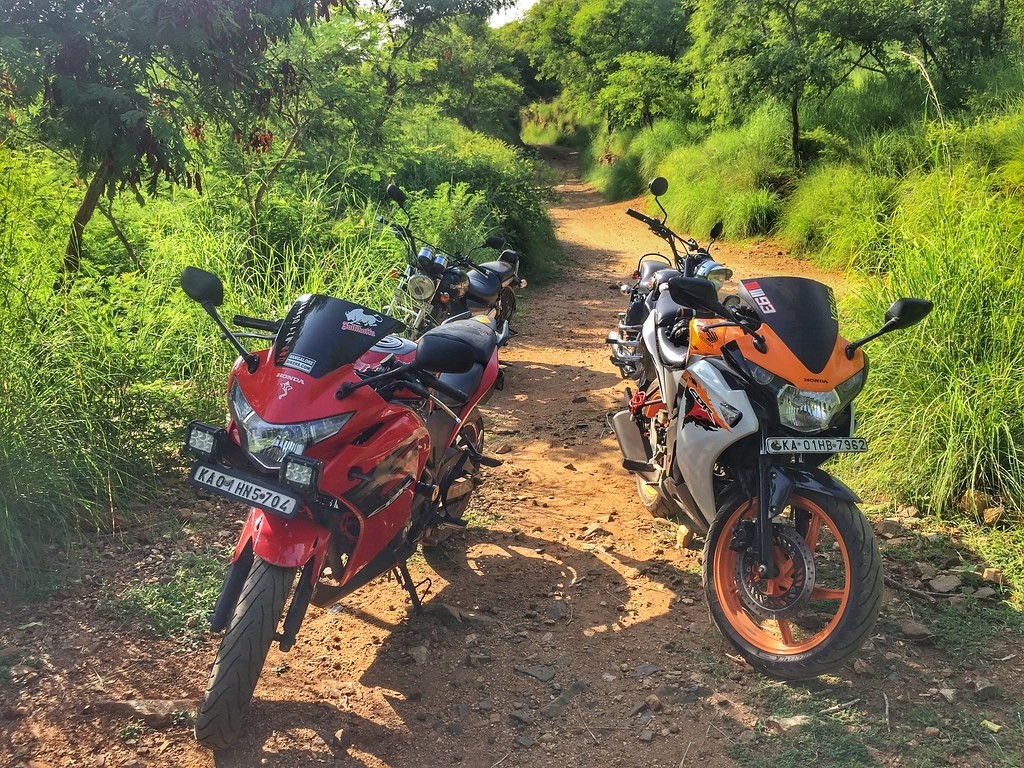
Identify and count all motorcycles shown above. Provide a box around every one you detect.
[376,184,527,368]
[607,177,733,381]
[613,275,940,683]
[179,265,509,754]
